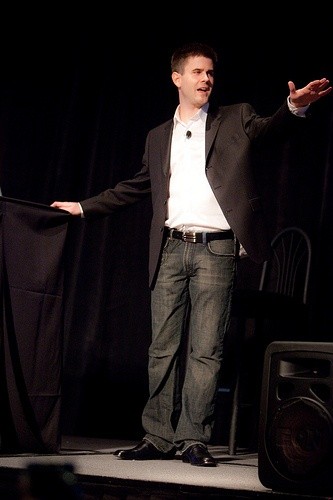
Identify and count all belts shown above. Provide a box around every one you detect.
[163,230,234,243]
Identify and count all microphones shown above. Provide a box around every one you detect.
[186,131,191,137]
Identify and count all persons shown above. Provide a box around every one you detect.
[50,43,332,467]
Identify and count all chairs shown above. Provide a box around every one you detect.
[218,225,313,457]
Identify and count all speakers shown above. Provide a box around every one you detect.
[258,342,333,497]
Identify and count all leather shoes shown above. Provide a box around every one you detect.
[112,439,176,460]
[182,444,218,466]
